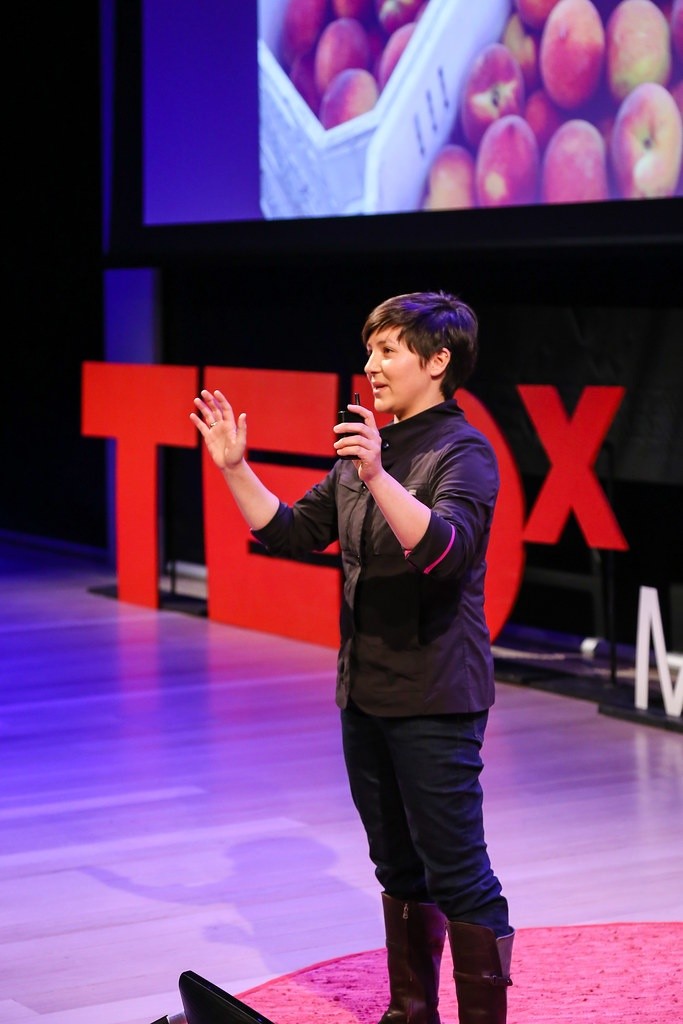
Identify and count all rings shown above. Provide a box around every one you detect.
[210,421,217,427]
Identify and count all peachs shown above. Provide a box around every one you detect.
[419,0,683,210]
[284,0,432,130]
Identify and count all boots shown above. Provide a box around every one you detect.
[445,920,515,1024]
[377,891,449,1024]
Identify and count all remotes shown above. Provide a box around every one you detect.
[337,392,365,460]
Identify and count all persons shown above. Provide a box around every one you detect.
[191,288,516,1024]
